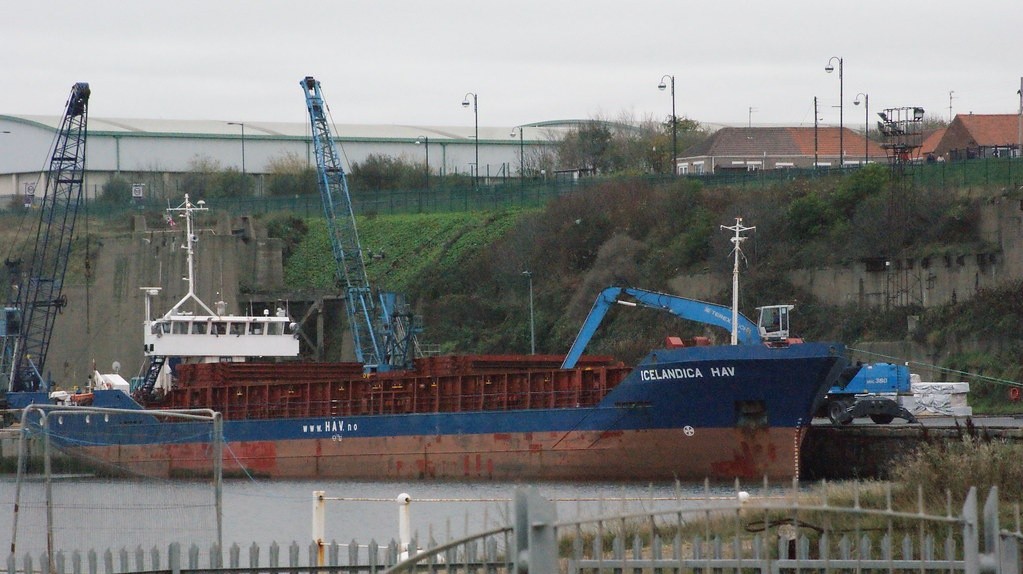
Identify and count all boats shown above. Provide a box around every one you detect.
[6,77,853,486]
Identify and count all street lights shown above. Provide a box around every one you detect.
[825,56,843,164]
[658,74,676,175]
[854,93,868,164]
[510,126,524,184]
[228,123,245,198]
[462,92,478,186]
[414,136,429,205]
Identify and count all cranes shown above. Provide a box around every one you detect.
[298,76,423,375]
[1,82,92,395]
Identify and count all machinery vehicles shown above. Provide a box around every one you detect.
[560,286,920,424]
